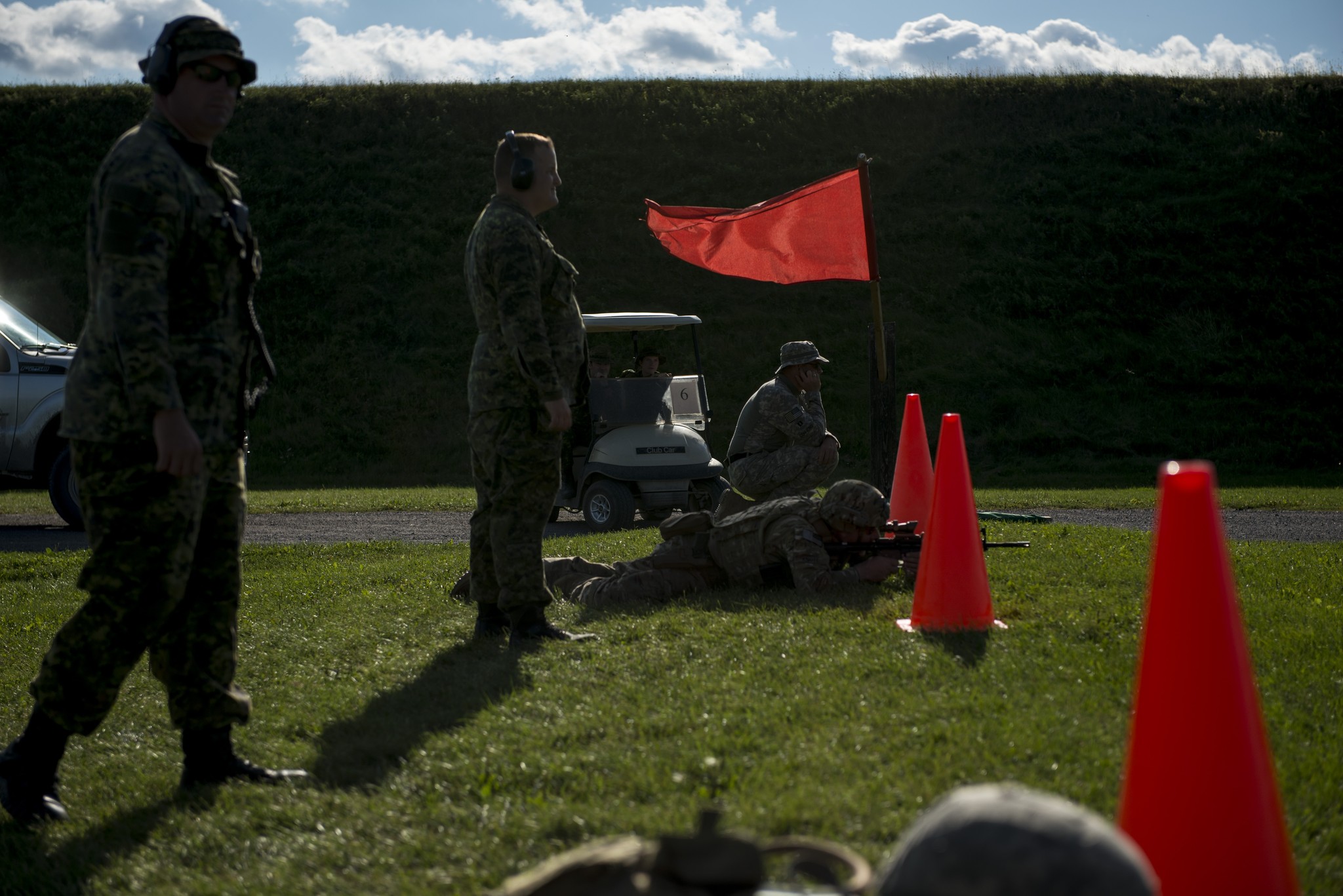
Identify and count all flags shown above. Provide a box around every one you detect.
[645,169,871,284]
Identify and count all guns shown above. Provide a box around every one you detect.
[830,521,1030,573]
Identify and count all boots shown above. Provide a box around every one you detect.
[475,605,511,637]
[715,489,765,522]
[0,712,73,824]
[508,605,601,652]
[180,725,308,790]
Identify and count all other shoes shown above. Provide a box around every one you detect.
[450,568,471,598]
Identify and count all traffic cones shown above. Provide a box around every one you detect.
[897,411,1016,636]
[1119,459,1305,895]
[886,393,941,549]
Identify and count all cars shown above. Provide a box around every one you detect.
[0,295,86,533]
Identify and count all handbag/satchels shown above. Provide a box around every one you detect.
[659,511,715,542]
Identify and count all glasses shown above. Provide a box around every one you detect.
[187,61,244,88]
[857,525,875,535]
[806,362,820,370]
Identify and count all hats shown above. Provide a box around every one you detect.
[146,16,256,86]
[620,370,635,378]
[635,347,667,366]
[775,341,829,373]
[588,345,614,364]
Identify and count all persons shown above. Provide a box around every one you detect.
[0,14,308,826]
[726,341,840,501]
[561,346,673,500]
[465,130,587,654]
[501,785,1159,896]
[451,479,920,611]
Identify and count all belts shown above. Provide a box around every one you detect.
[723,452,752,468]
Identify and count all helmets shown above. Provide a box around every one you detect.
[819,479,891,532]
[872,781,1159,896]
